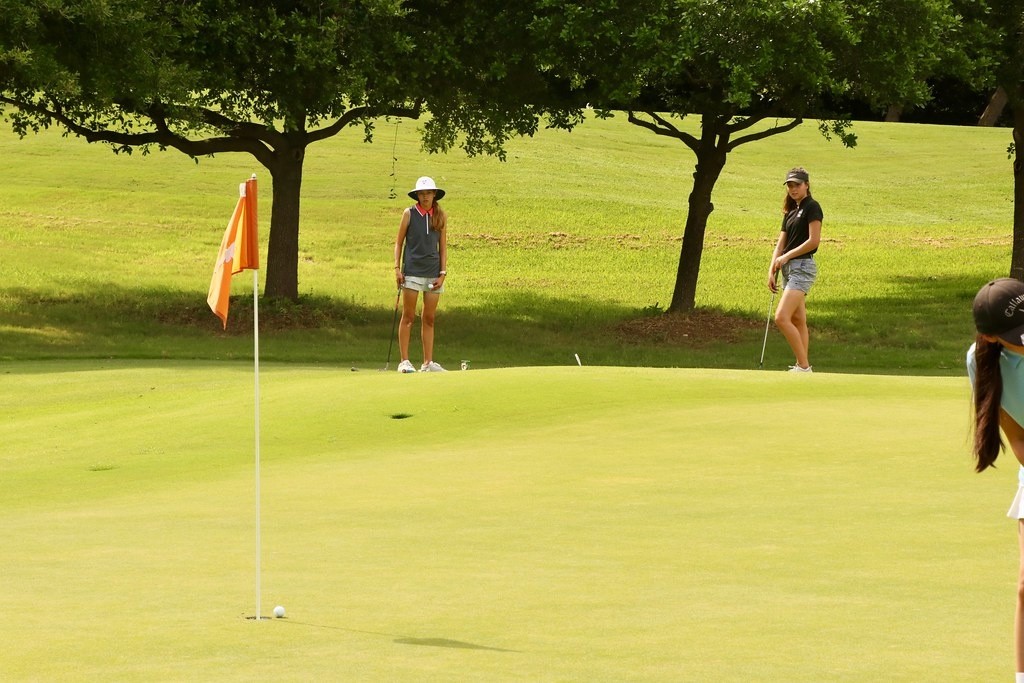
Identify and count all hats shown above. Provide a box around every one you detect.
[783,171,809,185]
[408,176,445,202]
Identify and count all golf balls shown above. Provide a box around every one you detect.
[273,606,285,618]
[427,284,434,289]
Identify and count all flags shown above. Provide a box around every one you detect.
[207,178,259,330]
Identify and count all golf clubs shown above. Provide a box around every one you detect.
[376,245,407,372]
[753,265,780,370]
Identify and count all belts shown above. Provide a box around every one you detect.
[795,255,813,259]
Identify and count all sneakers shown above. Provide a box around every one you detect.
[788,363,812,371]
[398,360,416,373]
[421,361,447,371]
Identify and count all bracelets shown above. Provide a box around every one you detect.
[395,267,400,269]
[440,271,447,276]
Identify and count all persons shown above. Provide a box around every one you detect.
[395,176,448,373]
[967,277,1024,683]
[767,167,823,373]
